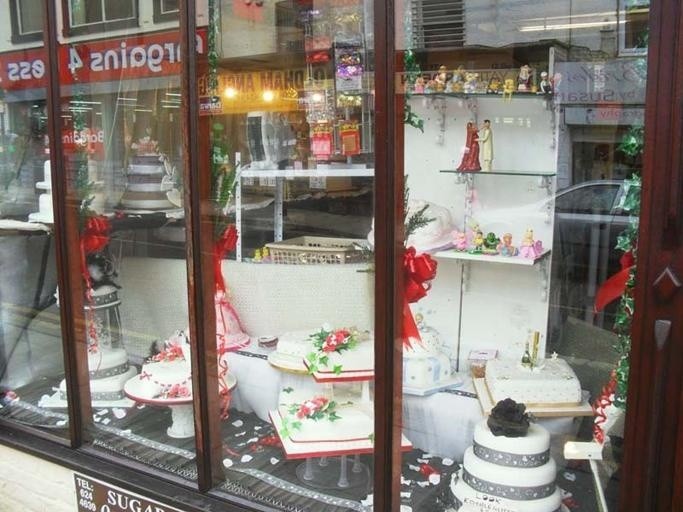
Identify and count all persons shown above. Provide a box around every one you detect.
[456,120,491,171]
[451,222,543,260]
[411,61,552,96]
[473,119,494,172]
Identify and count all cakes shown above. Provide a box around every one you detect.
[306,323,374,373]
[61,348,137,402]
[449,397,571,511]
[119,140,177,210]
[367,198,463,257]
[140,356,193,398]
[484,356,583,408]
[39,158,107,215]
[278,395,374,442]
[273,328,312,368]
[401,314,451,388]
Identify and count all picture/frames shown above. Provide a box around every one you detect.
[61,0,139,38]
[9,0,44,44]
[153,0,181,23]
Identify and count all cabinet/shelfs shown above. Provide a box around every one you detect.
[409,85,555,265]
[233,152,375,264]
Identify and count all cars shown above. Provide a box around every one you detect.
[469,177,660,279]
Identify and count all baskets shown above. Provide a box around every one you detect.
[265,236,371,265]
